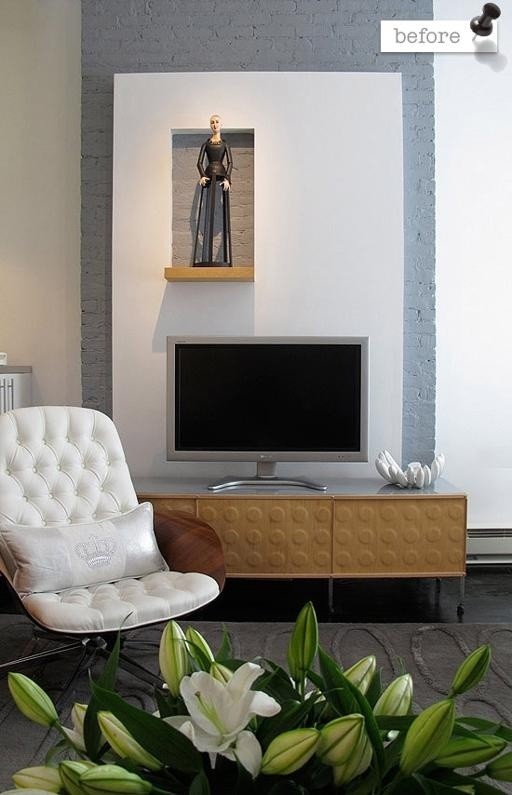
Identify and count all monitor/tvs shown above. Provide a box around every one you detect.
[166,335,370,491]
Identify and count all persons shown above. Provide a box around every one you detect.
[193,115,235,267]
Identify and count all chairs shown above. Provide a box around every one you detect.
[0,404,227,715]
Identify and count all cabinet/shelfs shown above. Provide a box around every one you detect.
[0,365,32,414]
[155,492,470,616]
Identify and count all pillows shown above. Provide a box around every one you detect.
[1,500,173,596]
